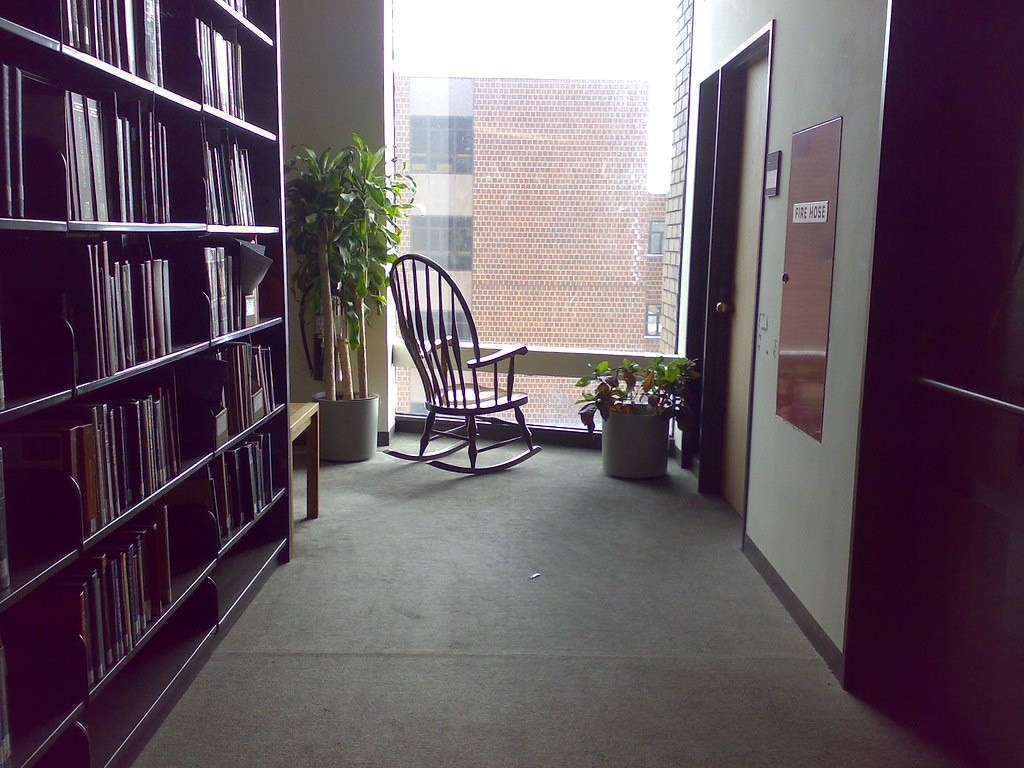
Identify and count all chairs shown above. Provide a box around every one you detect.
[383,253,545,474]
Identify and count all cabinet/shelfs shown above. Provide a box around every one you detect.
[0,0,291,768]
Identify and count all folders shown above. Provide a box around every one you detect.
[84,97,108,222]
[70,91,95,222]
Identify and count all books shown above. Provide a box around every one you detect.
[82,0,275,692]
[0,66,25,767]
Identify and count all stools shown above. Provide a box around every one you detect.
[288,402,323,560]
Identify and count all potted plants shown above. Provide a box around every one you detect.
[574,355,701,480]
[283,133,419,464]
